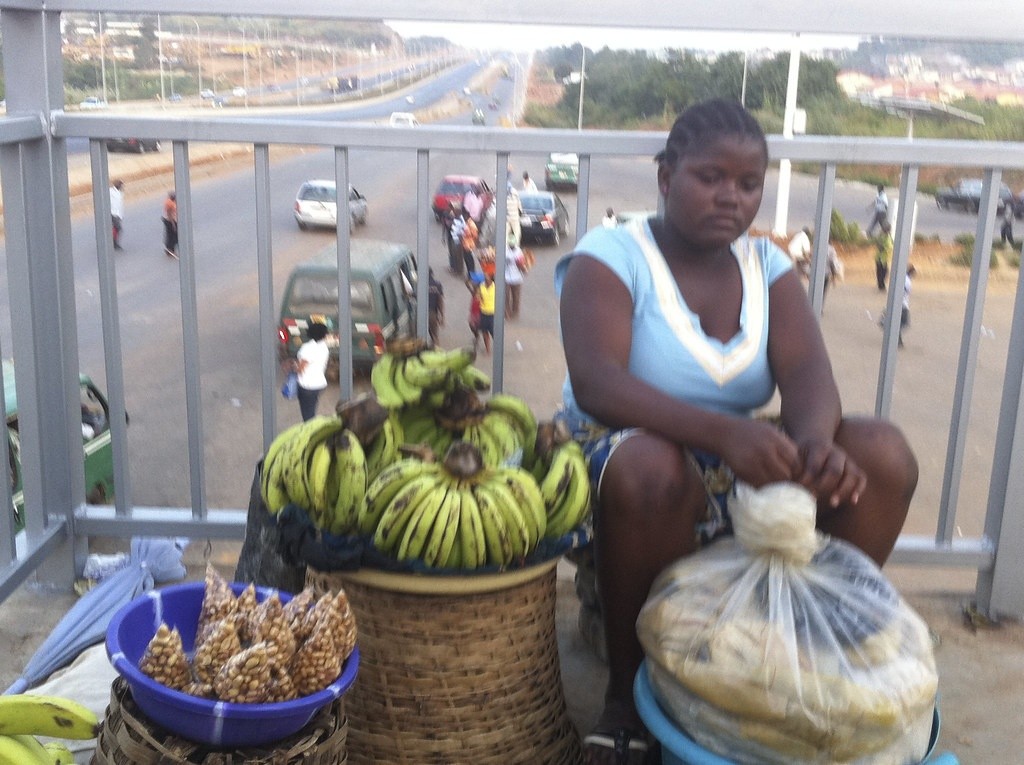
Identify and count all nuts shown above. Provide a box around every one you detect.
[137,568,357,704]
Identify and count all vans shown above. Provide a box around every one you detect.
[3,358,130,532]
[390,112,418,127]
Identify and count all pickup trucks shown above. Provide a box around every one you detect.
[78,97,105,109]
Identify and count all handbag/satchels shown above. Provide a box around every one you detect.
[282,370,298,401]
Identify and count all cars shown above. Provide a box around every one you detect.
[106,137,160,153]
[462,87,499,125]
[520,191,569,246]
[201,87,246,106]
[432,174,493,221]
[169,94,181,102]
[294,179,368,232]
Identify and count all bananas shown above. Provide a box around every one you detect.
[0,694,99,765]
[256,342,595,573]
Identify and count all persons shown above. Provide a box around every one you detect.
[545,101,920,765]
[602,208,616,227]
[162,192,179,259]
[293,322,329,424]
[110,181,124,250]
[822,239,840,316]
[898,264,916,349]
[1001,199,1015,249]
[788,227,812,283]
[427,167,538,356]
[866,183,888,237]
[874,222,894,293]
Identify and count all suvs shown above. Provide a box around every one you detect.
[935,179,1024,218]
[279,237,420,368]
[546,152,579,188]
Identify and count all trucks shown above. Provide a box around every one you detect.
[326,76,358,93]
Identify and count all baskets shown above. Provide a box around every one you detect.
[303,559,588,765]
[91,680,348,765]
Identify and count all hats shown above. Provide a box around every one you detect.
[468,271,484,285]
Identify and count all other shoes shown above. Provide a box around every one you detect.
[113,243,122,250]
[163,250,177,260]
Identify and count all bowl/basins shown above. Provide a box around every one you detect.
[103,583,360,750]
[632,660,939,765]
